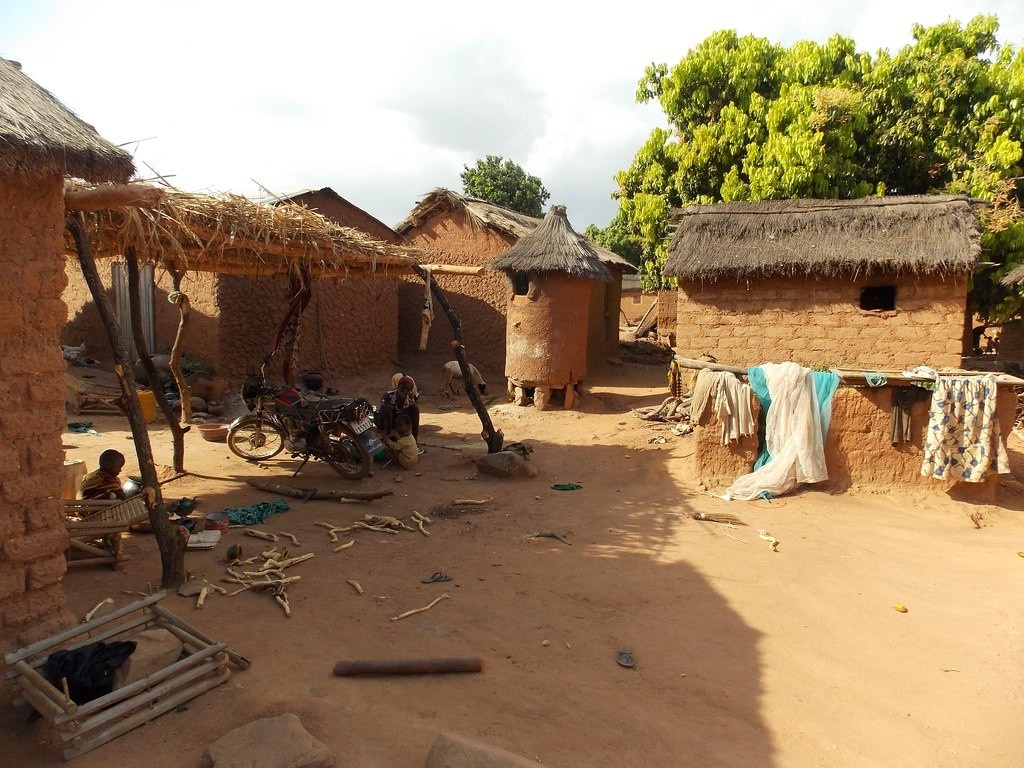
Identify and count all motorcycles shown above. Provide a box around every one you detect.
[226,352,386,481]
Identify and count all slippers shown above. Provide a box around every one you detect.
[421,572,453,583]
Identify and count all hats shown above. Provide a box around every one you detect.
[397,376,414,391]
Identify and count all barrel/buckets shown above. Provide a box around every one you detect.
[136,390,157,424]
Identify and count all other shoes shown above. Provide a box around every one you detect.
[417,445,428,453]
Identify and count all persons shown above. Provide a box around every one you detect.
[74,449,127,520]
[377,376,419,443]
[383,414,419,471]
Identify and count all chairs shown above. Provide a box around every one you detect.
[63,492,149,571]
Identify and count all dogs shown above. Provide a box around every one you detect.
[442,360,486,396]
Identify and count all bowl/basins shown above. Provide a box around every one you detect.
[130,519,153,534]
[205,512,228,534]
[196,423,230,442]
